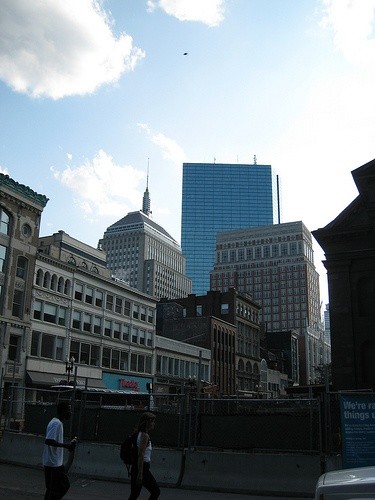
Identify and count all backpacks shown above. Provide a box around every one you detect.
[119,431,150,464]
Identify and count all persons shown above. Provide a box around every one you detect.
[42,399,78,500]
[128,412,161,500]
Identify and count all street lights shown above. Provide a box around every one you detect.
[64,355,75,384]
